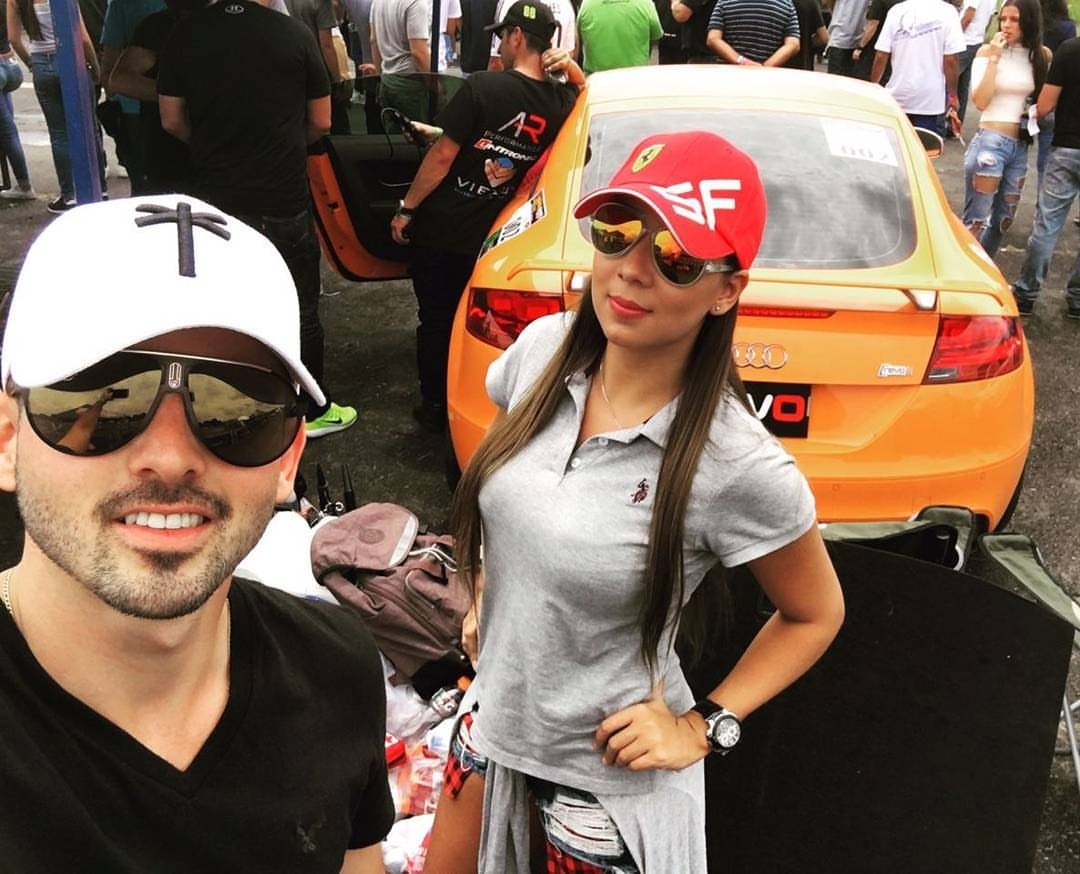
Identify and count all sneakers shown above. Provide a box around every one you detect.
[1,185,36,200]
[48,193,77,214]
[305,401,358,440]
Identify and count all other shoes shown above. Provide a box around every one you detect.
[118,164,130,179]
[1067,307,1080,319]
[413,398,445,434]
[105,167,111,180]
[1009,284,1034,314]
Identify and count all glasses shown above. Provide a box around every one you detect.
[589,202,738,288]
[11,344,311,466]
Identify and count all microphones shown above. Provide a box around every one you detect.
[400,114,429,148]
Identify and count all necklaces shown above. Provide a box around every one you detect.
[600,357,626,430]
[4,566,15,615]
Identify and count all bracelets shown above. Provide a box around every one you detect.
[396,214,412,219]
[737,55,743,64]
[989,56,998,64]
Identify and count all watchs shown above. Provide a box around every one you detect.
[690,695,743,756]
[395,199,416,216]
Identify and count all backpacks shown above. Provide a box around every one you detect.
[310,503,476,688]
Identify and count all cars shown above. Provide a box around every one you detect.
[304,64,1034,569]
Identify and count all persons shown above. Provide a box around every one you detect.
[963,0,1080,320]
[424,133,845,874]
[97,0,357,437]
[0,0,999,212]
[0,195,396,874]
[391,0,587,434]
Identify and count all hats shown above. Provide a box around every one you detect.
[574,132,766,270]
[483,0,562,49]
[1,192,326,406]
